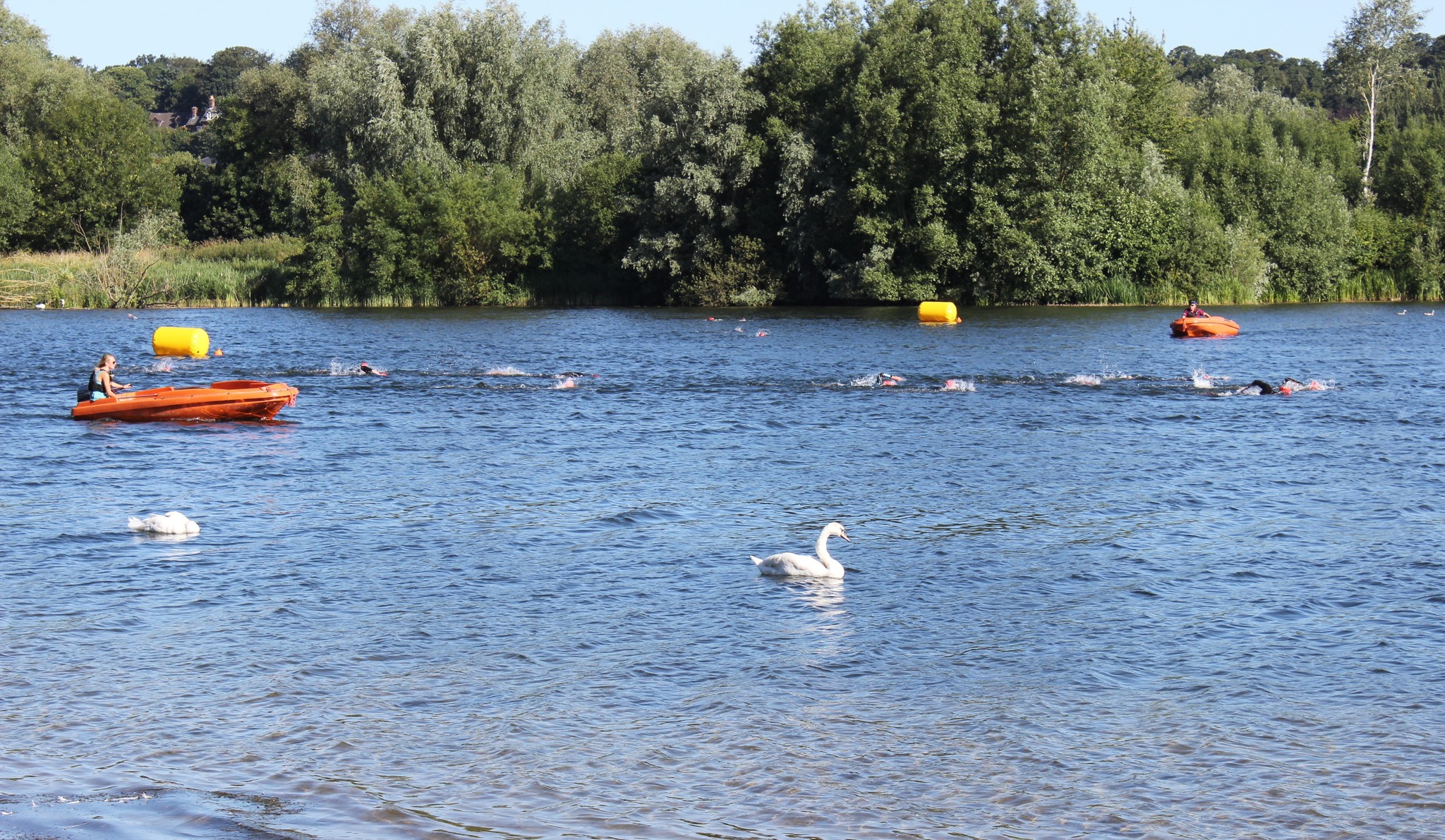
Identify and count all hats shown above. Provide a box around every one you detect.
[1189,300,1198,305]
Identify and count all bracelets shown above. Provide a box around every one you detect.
[122,385,124,389]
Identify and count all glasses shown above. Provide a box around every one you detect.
[107,361,116,364]
[1189,305,1195,306]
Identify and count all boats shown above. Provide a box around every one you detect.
[1169,316,1240,338]
[71,379,300,422]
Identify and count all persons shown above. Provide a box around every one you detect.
[1182,301,1211,319]
[543,372,602,379]
[361,363,387,375]
[759,332,769,336]
[1241,379,1291,396]
[709,317,714,321]
[1283,377,1324,392]
[88,354,132,401]
[853,373,902,388]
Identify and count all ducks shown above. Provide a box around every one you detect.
[1395,309,1407,315]
[1423,310,1435,315]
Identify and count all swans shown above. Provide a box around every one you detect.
[128,511,200,534]
[750,522,850,580]
